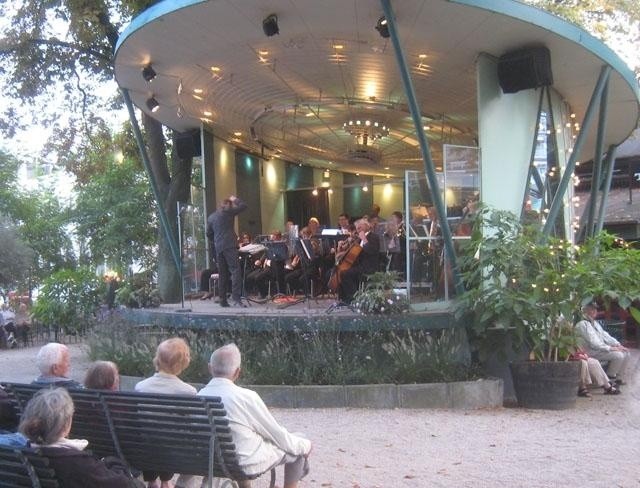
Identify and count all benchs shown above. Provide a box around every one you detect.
[600,360,610,367]
[0,381,276,488]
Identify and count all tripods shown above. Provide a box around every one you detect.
[325,270,357,314]
[295,258,318,305]
[239,254,262,306]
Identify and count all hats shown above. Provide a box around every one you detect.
[587,301,601,308]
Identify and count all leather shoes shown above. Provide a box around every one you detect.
[234,301,247,308]
[220,300,230,307]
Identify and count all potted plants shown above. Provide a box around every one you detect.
[451,200,640,413]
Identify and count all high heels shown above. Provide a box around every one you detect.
[200,295,211,300]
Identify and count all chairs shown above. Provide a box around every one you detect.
[208,216,471,313]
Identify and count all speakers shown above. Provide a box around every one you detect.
[497,47,554,93]
[176,129,201,159]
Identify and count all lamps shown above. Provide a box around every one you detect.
[145,97,161,113]
[262,14,280,38]
[141,66,158,83]
[374,16,391,39]
[340,116,391,149]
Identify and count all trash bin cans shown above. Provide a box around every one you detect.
[597,319,626,347]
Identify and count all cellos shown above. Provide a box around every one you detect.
[327,221,378,289]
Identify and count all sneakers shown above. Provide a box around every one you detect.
[7,336,18,349]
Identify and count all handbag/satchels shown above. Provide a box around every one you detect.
[568,352,589,360]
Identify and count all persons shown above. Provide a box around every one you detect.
[572,294,631,385]
[198,341,312,488]
[552,309,621,398]
[29,342,78,389]
[84,359,172,488]
[137,338,199,488]
[243,191,481,306]
[104,275,118,311]
[18,388,145,488]
[207,196,248,308]
[0,292,31,348]
[200,268,217,300]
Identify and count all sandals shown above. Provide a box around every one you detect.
[579,388,592,398]
[603,386,621,395]
[609,377,625,388]
